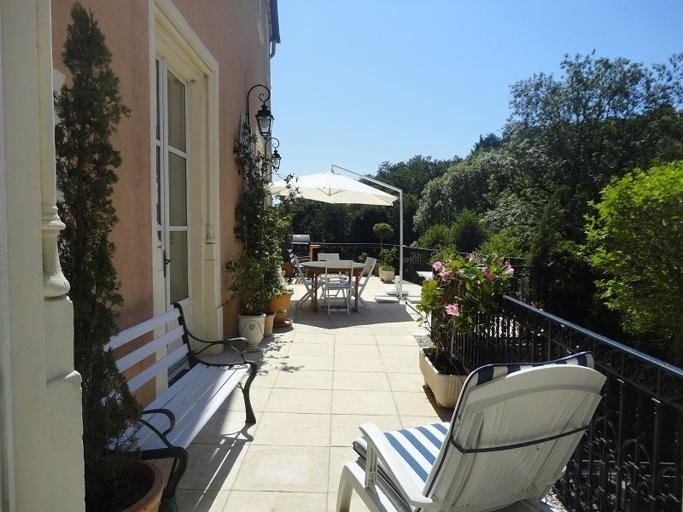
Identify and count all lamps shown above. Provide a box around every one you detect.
[245,84,275,139]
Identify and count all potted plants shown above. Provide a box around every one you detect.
[233,121,301,352]
[54,2,164,512]
[372,222,395,276]
[379,246,398,281]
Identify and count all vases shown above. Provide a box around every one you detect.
[419,346,468,408]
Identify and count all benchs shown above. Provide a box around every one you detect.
[101,301,258,512]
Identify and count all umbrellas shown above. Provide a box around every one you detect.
[263,165,404,300]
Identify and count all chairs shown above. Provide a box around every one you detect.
[336,348,608,512]
[294,253,377,317]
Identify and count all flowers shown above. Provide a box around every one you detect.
[417,248,515,361]
